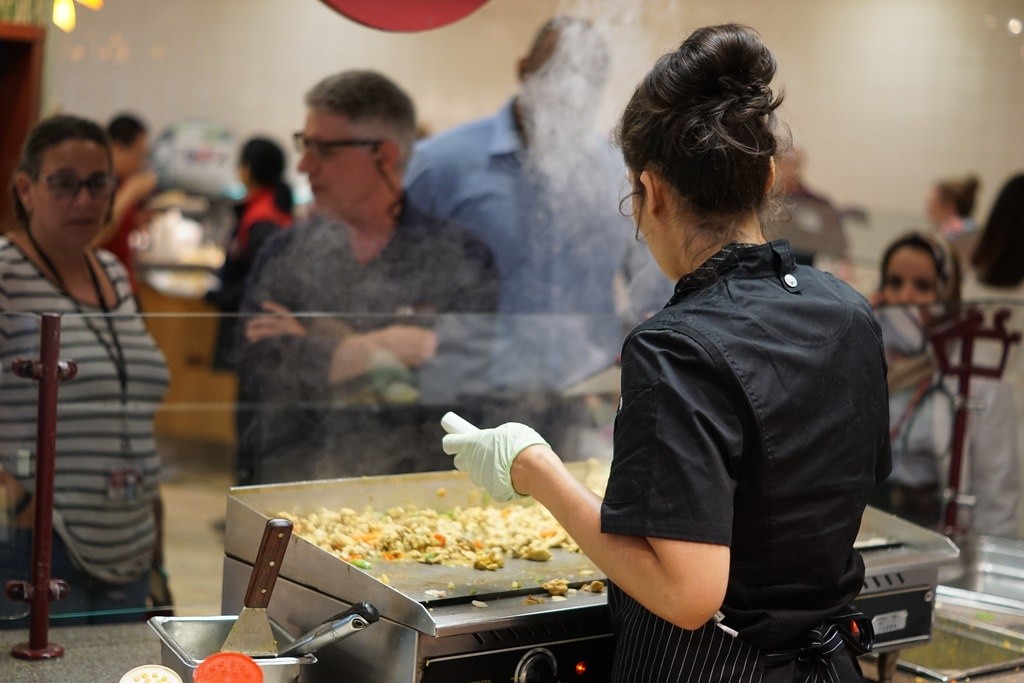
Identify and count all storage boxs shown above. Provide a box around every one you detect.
[147,614,317,683]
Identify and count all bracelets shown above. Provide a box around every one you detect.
[6,492,32,519]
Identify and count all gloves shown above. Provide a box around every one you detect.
[442,422,551,508]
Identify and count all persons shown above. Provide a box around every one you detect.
[93,115,160,288]
[398,18,674,466]
[877,229,963,305]
[762,150,847,257]
[199,139,293,529]
[227,71,498,491]
[0,117,173,621]
[441,24,892,682]
[928,178,978,240]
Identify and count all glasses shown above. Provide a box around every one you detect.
[31,168,117,200]
[293,133,379,160]
